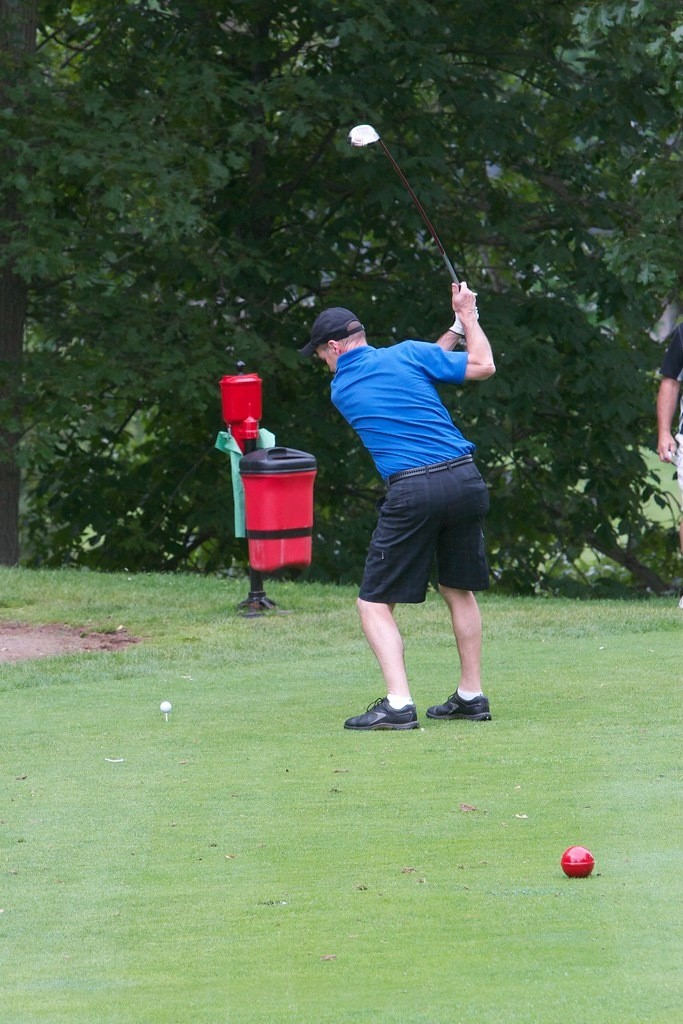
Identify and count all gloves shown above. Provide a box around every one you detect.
[449,293,479,335]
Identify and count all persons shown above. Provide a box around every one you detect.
[656,321,683,608]
[297,281,497,730]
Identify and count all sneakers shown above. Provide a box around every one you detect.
[344,696,419,730]
[426,686,492,721]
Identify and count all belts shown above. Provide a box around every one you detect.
[389,454,473,484]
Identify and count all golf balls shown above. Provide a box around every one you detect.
[159,702,173,712]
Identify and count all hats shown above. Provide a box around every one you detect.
[302,307,365,355]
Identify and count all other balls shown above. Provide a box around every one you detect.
[561,845,595,879]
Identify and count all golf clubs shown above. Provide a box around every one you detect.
[346,124,461,287]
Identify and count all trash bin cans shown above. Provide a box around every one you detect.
[238,446,318,573]
